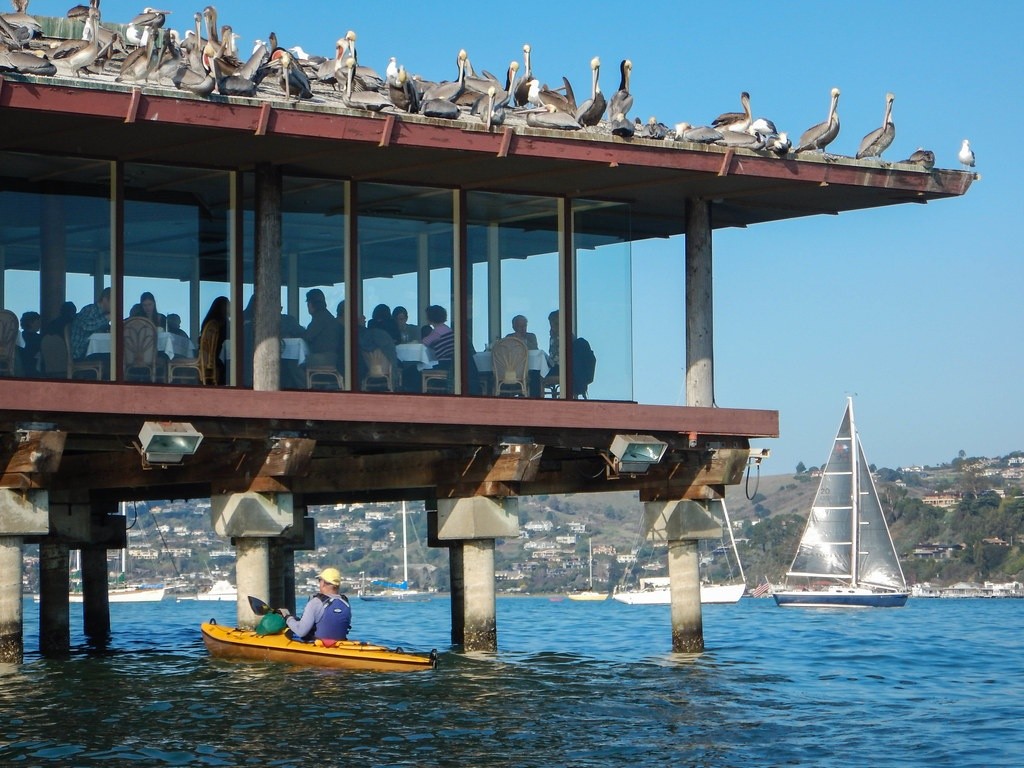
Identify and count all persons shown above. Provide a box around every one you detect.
[71,287,111,380]
[280,567,351,641]
[19,301,76,363]
[129,292,188,337]
[530,310,560,398]
[506,315,538,350]
[200,296,230,346]
[359,304,454,392]
[289,288,345,388]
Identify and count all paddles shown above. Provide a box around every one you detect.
[247,596,300,620]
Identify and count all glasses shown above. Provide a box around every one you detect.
[319,578,322,582]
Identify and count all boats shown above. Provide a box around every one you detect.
[195,580,238,602]
[201,618,438,671]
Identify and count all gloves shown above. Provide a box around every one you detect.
[277,608,291,617]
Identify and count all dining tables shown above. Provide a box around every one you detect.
[219,337,310,391]
[473,349,553,399]
[395,339,435,393]
[85,332,194,386]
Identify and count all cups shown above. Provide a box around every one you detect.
[401,331,409,341]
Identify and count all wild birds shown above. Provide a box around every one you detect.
[608,59,793,154]
[958,139,977,170]
[899,148,934,169]
[385,46,606,130]
[856,92,897,167]
[795,88,841,160]
[0,0,397,113]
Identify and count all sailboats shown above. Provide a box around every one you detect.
[613,498,746,604]
[68,501,165,602]
[359,501,439,603]
[773,397,911,607]
[568,538,609,600]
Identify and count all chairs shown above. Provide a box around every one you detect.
[0,309,595,399]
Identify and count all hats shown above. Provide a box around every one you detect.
[315,568,340,586]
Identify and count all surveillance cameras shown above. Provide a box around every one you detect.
[748,447,770,458]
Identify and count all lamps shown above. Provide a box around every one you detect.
[131,422,204,471]
[599,434,668,480]
[688,432,697,448]
